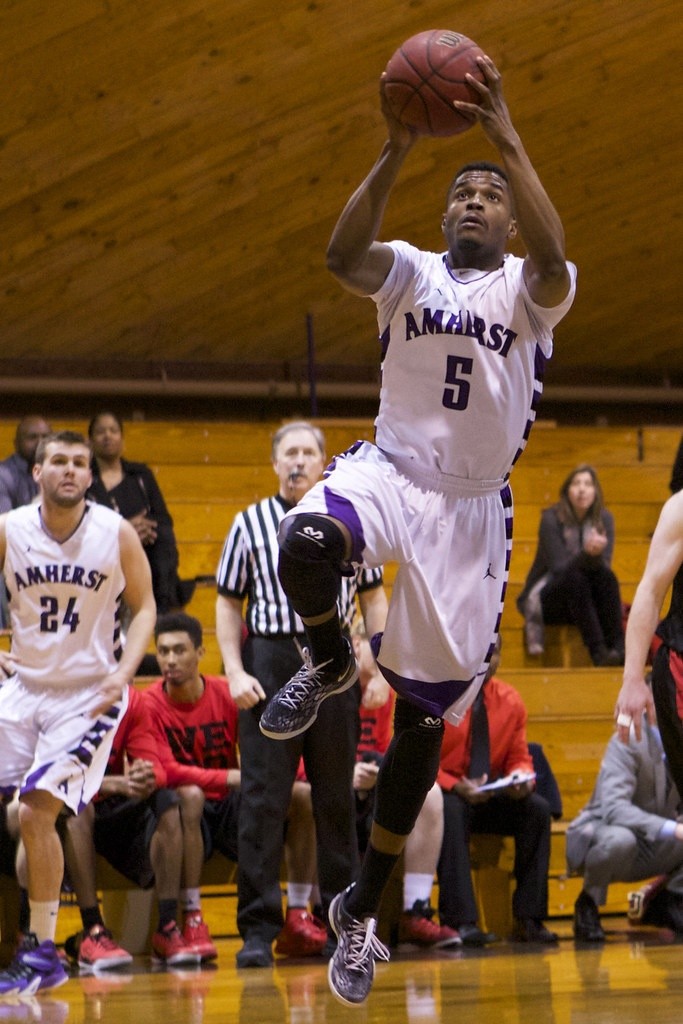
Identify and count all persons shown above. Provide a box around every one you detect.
[259,55,578,1006]
[0,408,683,1002]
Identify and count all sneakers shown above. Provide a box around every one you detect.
[258,635,357,741]
[0,931,68,1001]
[328,881,391,1008]
[400,898,462,948]
[276,904,328,958]
[77,923,134,970]
[182,909,219,963]
[150,919,201,965]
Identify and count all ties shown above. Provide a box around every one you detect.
[469,690,490,782]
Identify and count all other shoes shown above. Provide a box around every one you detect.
[236,935,272,967]
[514,916,556,944]
[457,923,495,946]
[640,885,683,934]
[572,897,605,940]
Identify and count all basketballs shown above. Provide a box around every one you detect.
[384,28,490,139]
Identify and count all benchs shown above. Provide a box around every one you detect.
[1,420,683,943]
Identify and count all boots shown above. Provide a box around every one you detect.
[582,626,627,667]
